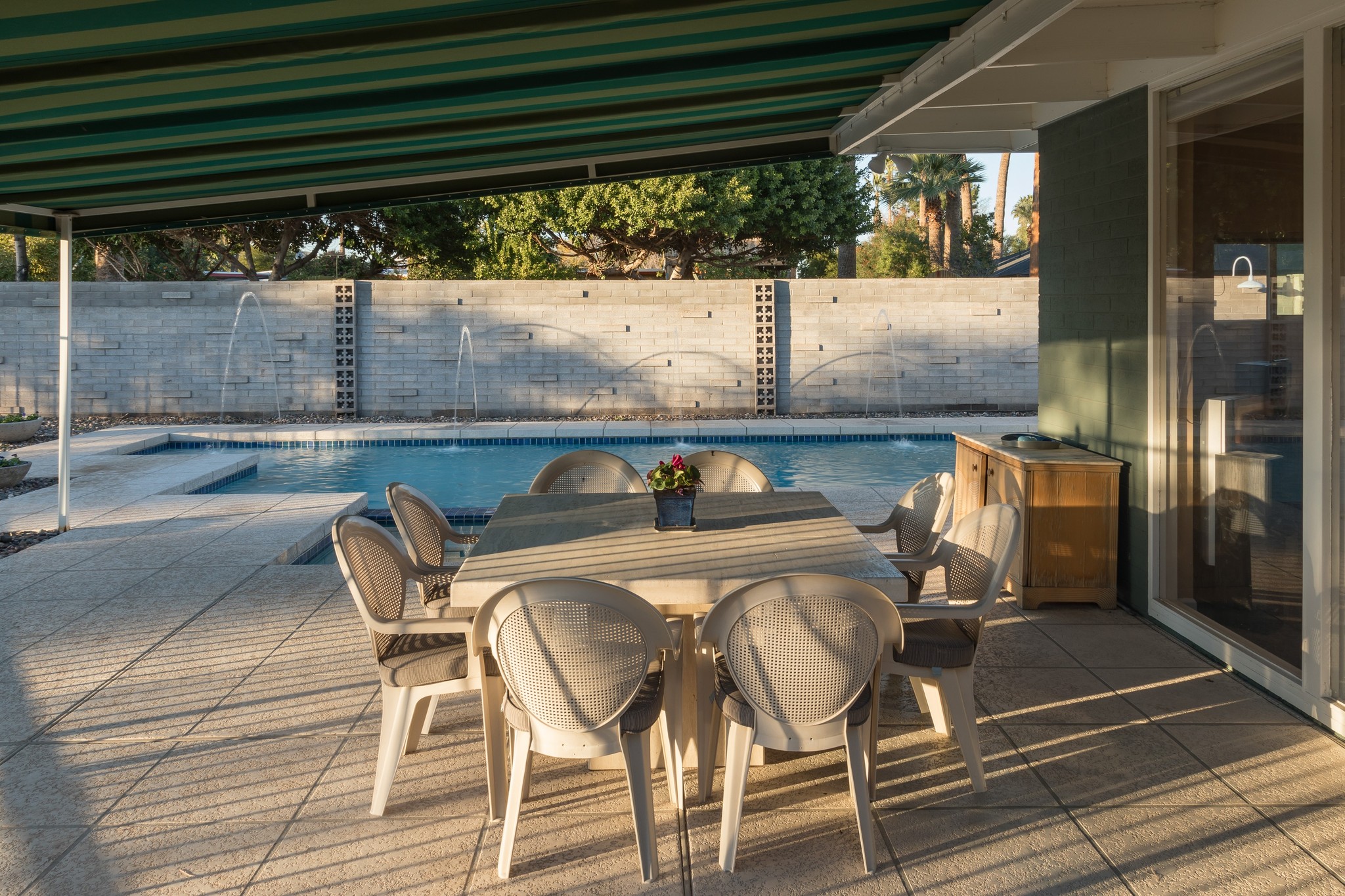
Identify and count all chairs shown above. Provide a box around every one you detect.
[386,481,481,619]
[683,449,775,493]
[694,574,905,875]
[527,447,650,494]
[862,502,1022,802]
[469,576,686,886]
[332,516,517,821]
[855,470,955,604]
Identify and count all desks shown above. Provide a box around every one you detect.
[449,491,907,770]
[951,431,1125,611]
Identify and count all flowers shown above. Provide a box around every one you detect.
[0,454,21,468]
[0,411,39,423]
[643,454,703,490]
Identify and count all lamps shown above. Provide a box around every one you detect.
[1231,256,1267,289]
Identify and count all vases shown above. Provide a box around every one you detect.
[0,416,43,444]
[0,461,33,489]
[652,485,698,532]
